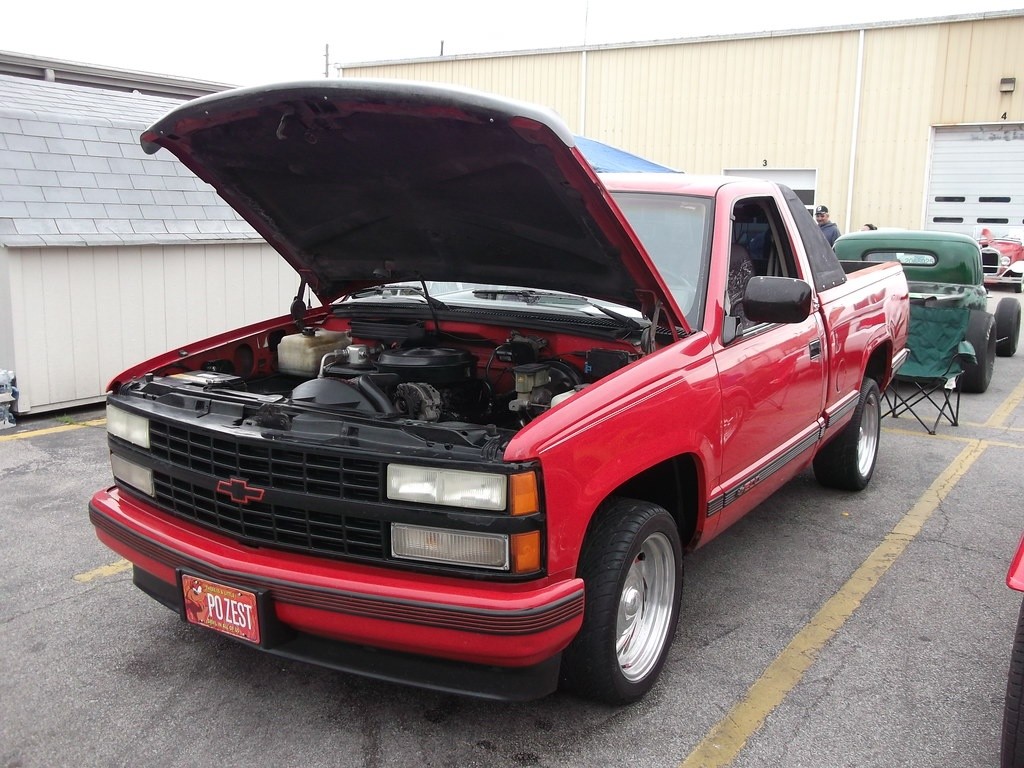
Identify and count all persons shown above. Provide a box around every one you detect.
[814,205,840,247]
[862,223,877,232]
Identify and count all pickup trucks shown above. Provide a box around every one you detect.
[86,78,912,714]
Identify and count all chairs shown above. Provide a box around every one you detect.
[879,304,979,434]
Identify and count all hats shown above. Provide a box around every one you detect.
[815,205,828,215]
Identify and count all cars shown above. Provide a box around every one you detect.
[827,228,1021,394]
[970,229,1024,292]
